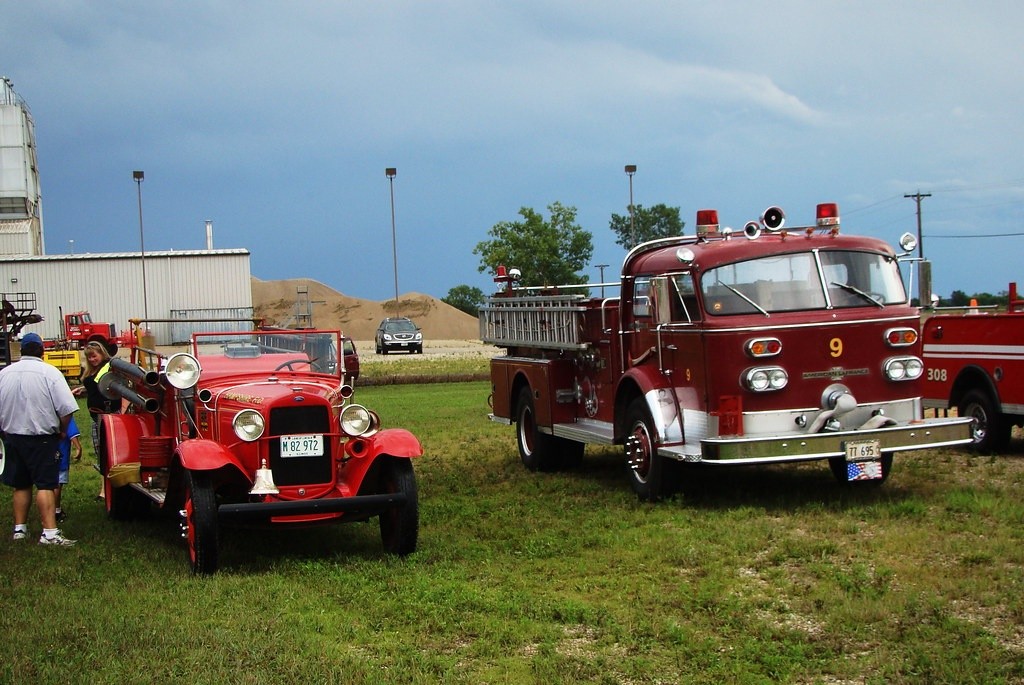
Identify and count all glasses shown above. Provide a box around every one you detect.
[84,342,100,347]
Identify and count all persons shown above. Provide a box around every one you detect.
[71,333,122,502]
[53,412,82,525]
[0,332,80,548]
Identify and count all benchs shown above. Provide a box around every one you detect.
[193,353,310,384]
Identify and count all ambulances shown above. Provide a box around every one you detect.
[907,281,1024,454]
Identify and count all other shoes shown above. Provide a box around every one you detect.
[14,529,31,541]
[55,510,67,522]
[37,532,79,547]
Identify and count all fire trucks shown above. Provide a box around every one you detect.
[477,199,985,506]
[92,313,427,570]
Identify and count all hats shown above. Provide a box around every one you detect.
[22,331,43,348]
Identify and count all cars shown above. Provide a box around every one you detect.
[9,299,356,392]
[375,317,423,356]
